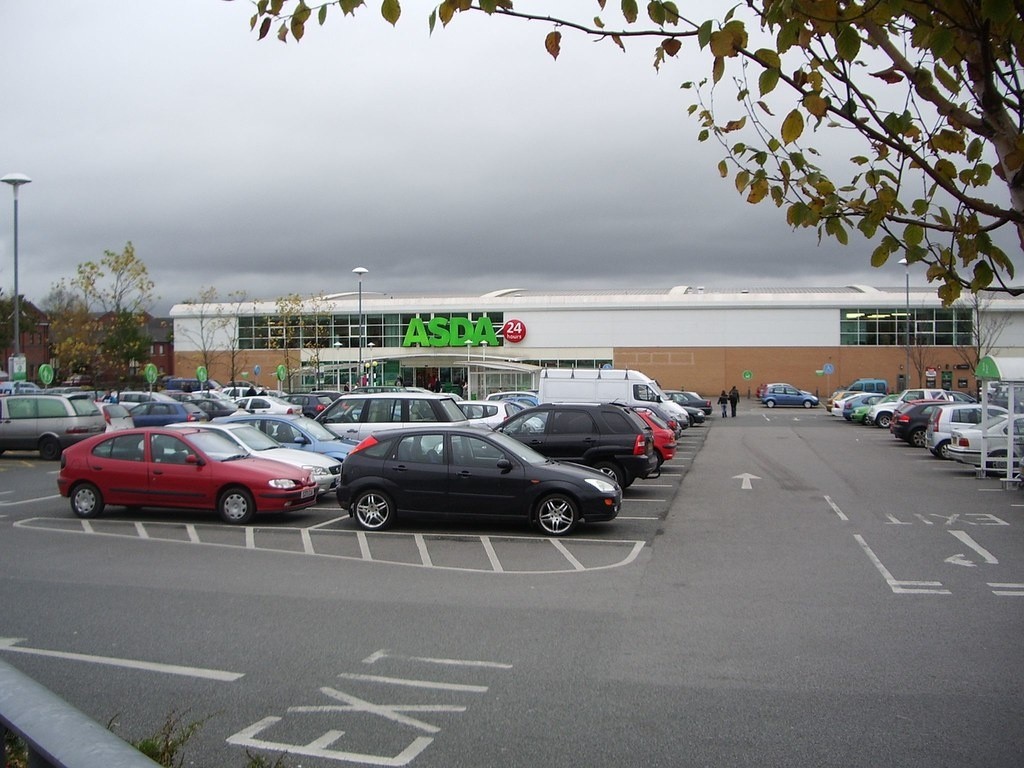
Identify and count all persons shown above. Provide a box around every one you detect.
[728,386,739,417]
[246,384,257,396]
[344,381,349,392]
[355,373,503,399]
[110,379,222,400]
[717,390,729,418]
[258,386,268,396]
[95,388,117,403]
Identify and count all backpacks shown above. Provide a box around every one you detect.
[731,390,737,400]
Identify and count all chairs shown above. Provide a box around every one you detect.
[152,444,169,463]
[170,440,189,464]
[277,424,291,442]
[427,448,442,463]
[410,405,422,420]
[967,412,978,424]
[267,425,275,437]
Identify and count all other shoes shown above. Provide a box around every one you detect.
[734,414,736,416]
[732,415,735,417]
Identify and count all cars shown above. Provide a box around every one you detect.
[831,391,883,421]
[947,414,1024,477]
[868,389,953,428]
[889,399,945,434]
[57,414,622,537]
[543,402,682,472]
[755,383,812,399]
[893,402,969,448]
[93,378,344,432]
[933,391,977,404]
[273,386,538,442]
[761,385,819,409]
[850,394,920,426]
[925,404,1008,460]
[842,395,887,421]
[650,390,713,427]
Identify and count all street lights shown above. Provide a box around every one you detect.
[464,339,488,401]
[0,173,32,356]
[333,267,376,392]
[897,258,918,388]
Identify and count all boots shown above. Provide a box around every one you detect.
[722,413,724,418]
[725,411,727,417]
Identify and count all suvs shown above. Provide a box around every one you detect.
[439,403,658,490]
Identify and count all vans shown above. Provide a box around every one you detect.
[0,393,107,459]
[847,379,889,396]
[538,363,691,429]
